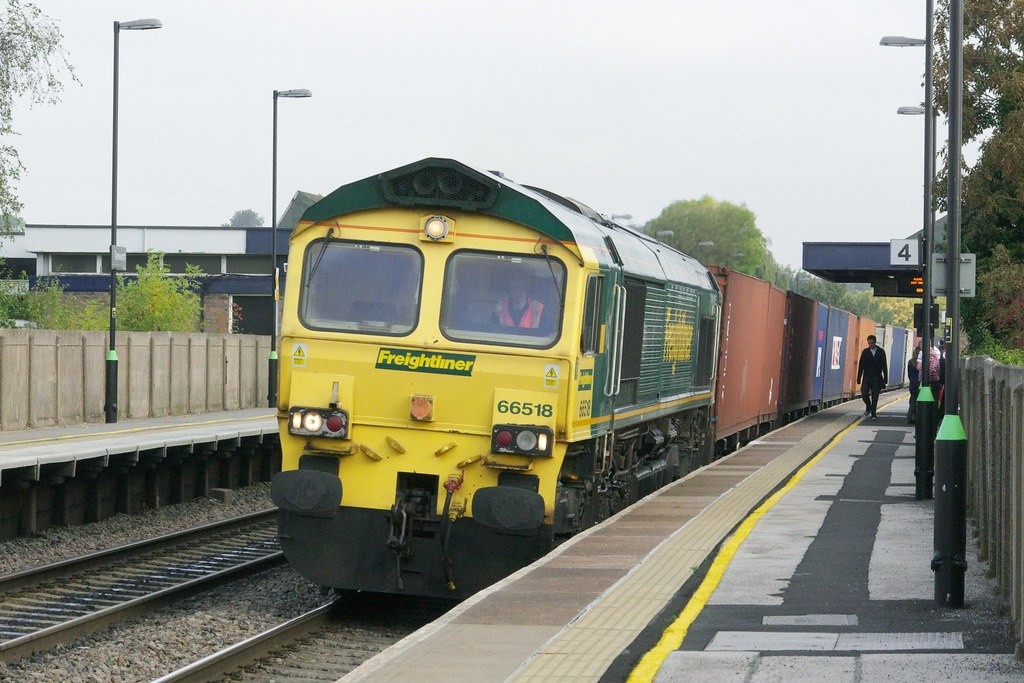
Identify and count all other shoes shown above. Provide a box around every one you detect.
[872,417,879,421]
[863,410,870,417]
[905,419,913,424]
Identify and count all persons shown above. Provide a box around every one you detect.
[488,273,547,329]
[907,335,944,423]
[857,335,887,420]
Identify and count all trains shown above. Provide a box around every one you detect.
[267,155,924,620]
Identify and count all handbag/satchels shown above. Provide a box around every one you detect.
[918,347,941,383]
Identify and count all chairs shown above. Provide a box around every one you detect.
[349,300,397,324]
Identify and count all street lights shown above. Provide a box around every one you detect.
[104,17,164,425]
[879,34,941,500]
[267,89,314,409]
